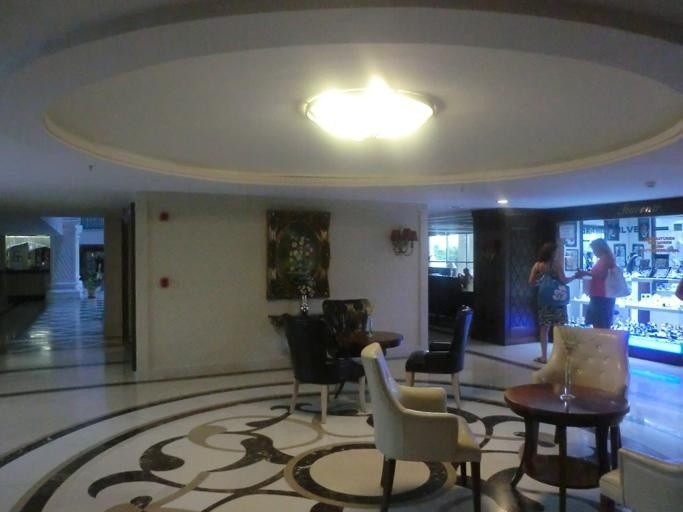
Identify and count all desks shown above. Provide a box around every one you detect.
[334,330,405,400]
[504,382,631,512]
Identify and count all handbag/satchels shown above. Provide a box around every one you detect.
[537,273,570,308]
[605,266,631,298]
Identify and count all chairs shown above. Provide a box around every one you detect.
[405,304,473,409]
[281,298,683,512]
[361,342,481,512]
[599,448,682,512]
[281,317,365,424]
[532,325,630,479]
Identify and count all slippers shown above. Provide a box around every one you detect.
[533,357,547,364]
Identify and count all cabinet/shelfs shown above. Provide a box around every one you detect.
[569,276,683,367]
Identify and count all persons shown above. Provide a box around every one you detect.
[527,242,583,364]
[576,240,617,330]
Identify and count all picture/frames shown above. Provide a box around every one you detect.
[614,243,672,278]
[560,221,579,272]
[264,208,331,300]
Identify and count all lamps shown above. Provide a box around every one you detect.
[388,225,419,257]
